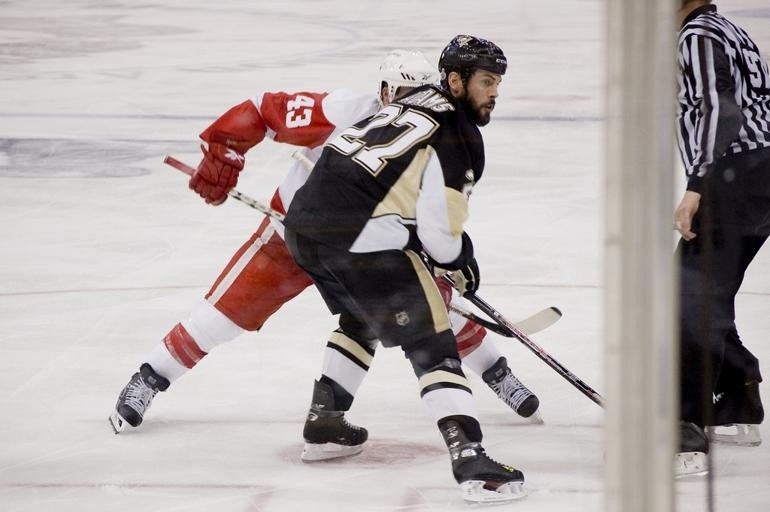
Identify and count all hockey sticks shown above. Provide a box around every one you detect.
[164,154,562,338]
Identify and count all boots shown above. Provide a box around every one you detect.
[482,357,538,417]
[438,420,523,484]
[303,378,368,445]
[116,363,170,427]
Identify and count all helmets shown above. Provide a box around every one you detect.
[438,35,507,96]
[376,49,442,109]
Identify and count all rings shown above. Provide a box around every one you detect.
[674,222,680,230]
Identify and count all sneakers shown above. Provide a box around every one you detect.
[707,380,763,426]
[679,421,709,453]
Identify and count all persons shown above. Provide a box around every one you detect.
[281,34,524,490]
[111,48,539,428]
[672,0,770,454]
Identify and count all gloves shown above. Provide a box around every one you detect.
[189,142,245,206]
[425,231,480,314]
